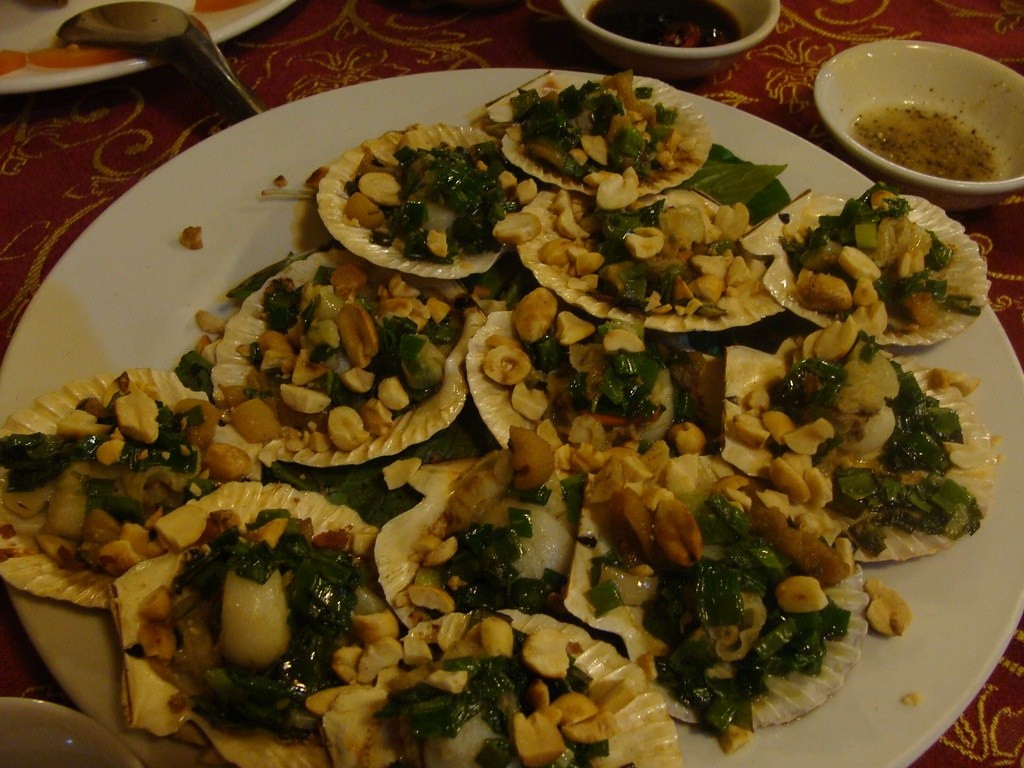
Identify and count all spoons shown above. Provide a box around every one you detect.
[57,0,269,123]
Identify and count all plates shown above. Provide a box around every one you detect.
[0,68,1023,766]
[559,1,781,76]
[0,1,295,95]
[0,696,141,767]
[814,40,1024,211]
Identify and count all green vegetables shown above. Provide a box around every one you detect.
[0,83,984,768]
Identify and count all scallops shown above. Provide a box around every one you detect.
[2,72,994,768]
